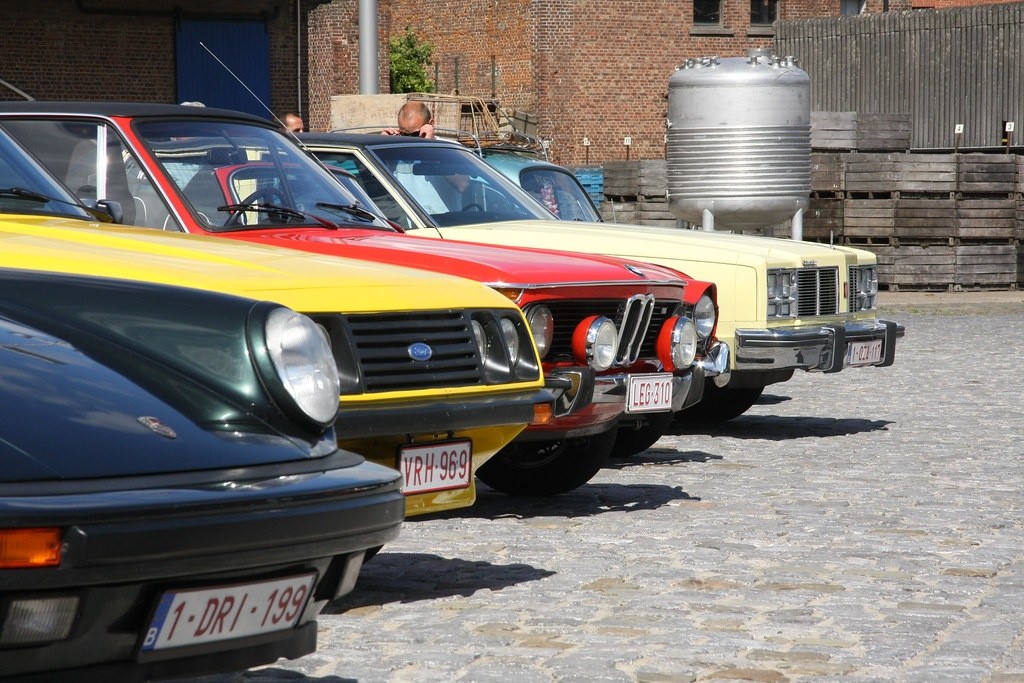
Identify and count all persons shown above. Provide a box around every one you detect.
[381,101,470,226]
[161,101,207,191]
[259,111,305,222]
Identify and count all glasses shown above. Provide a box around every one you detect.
[399,130,420,137]
[294,128,309,132]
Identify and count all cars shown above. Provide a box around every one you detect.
[283,130,910,421]
[4,99,730,500]
[0,266,410,683]
[474,146,605,225]
[0,127,571,520]
